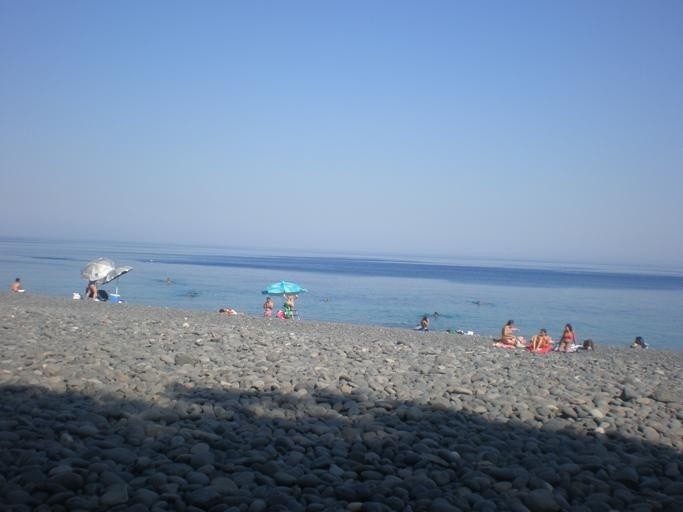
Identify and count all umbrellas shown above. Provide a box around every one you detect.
[99,264,133,286]
[261,279,308,296]
[79,255,116,281]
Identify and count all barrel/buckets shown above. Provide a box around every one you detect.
[109,293,121,304]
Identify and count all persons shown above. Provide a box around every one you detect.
[263,297,273,317]
[631,336,648,350]
[531,327,553,351]
[501,319,520,345]
[557,323,576,353]
[9,277,21,291]
[217,308,243,315]
[84,280,98,302]
[282,295,298,318]
[420,316,428,329]
